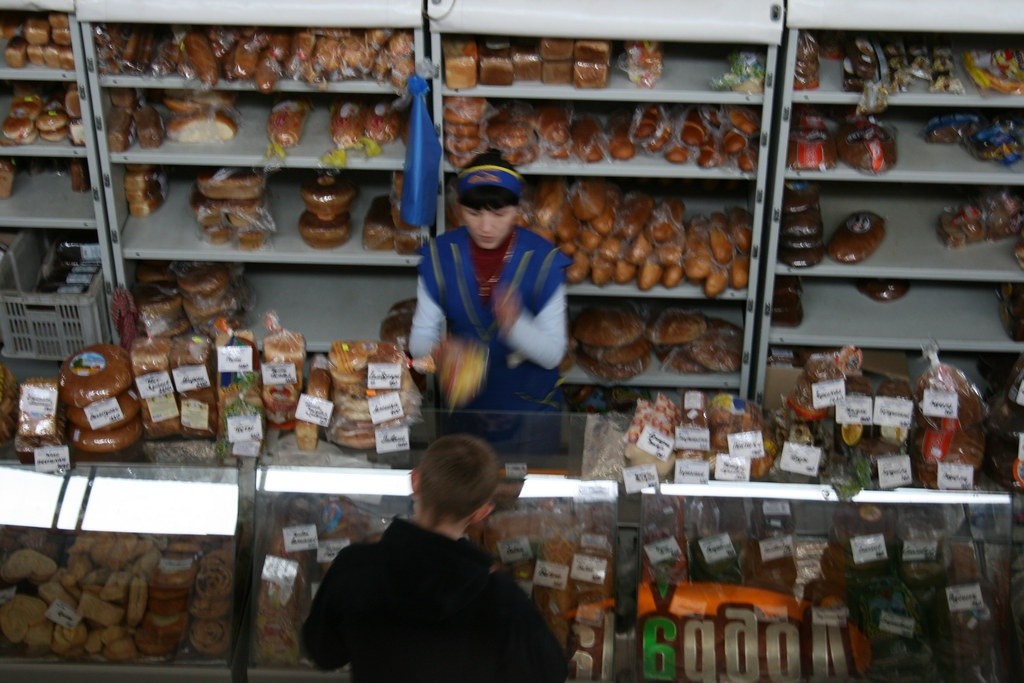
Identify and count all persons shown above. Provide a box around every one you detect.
[408,152,572,454]
[302,434,571,683]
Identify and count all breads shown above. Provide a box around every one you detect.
[0,503,620,668]
[0,8,1024,493]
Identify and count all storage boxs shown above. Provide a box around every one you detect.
[1,260,113,361]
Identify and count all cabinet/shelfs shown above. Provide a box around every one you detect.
[0,0,122,371]
[422,0,786,422]
[74,0,432,378]
[752,0,1024,428]
